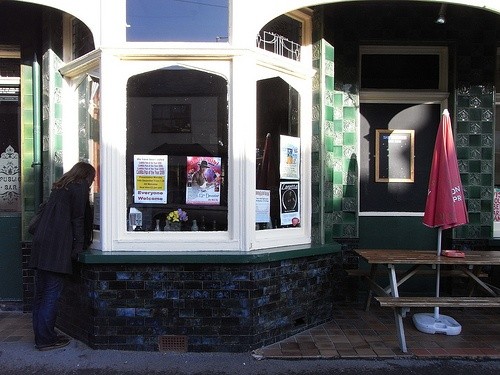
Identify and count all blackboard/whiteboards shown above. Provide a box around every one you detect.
[375,129,415,183]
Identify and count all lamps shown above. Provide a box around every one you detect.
[436,4,448,23]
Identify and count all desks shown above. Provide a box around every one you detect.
[353,249,500,353]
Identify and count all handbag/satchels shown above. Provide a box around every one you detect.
[27,206,47,234]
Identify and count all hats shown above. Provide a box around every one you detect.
[198,161,210,168]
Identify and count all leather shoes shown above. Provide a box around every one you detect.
[38,336,70,351]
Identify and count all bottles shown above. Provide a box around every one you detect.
[155,220,160,231]
[135,226,141,230]
[198,216,217,231]
[186,219,198,231]
[164,220,170,231]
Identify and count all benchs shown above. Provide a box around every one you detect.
[346,270,500,306]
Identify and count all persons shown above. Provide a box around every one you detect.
[191,160,212,188]
[30,161,97,351]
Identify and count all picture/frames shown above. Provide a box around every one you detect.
[375,129,415,183]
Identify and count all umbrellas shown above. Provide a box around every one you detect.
[422,110,471,318]
[258,132,283,230]
